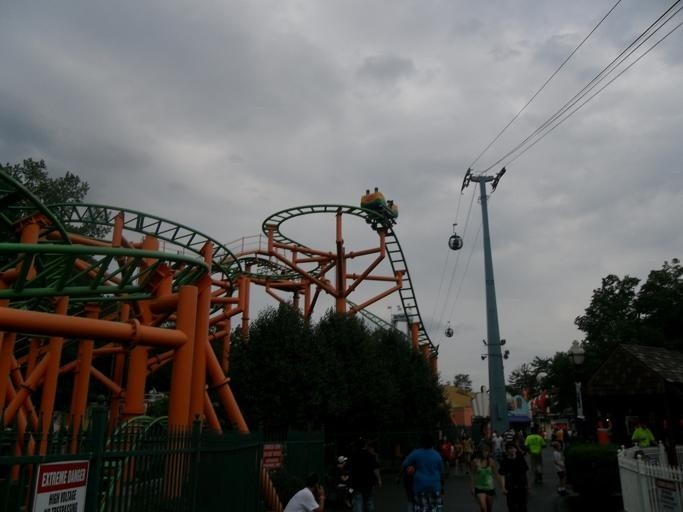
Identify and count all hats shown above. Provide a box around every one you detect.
[337,455,348,464]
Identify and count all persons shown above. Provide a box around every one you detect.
[280,413,683,512]
